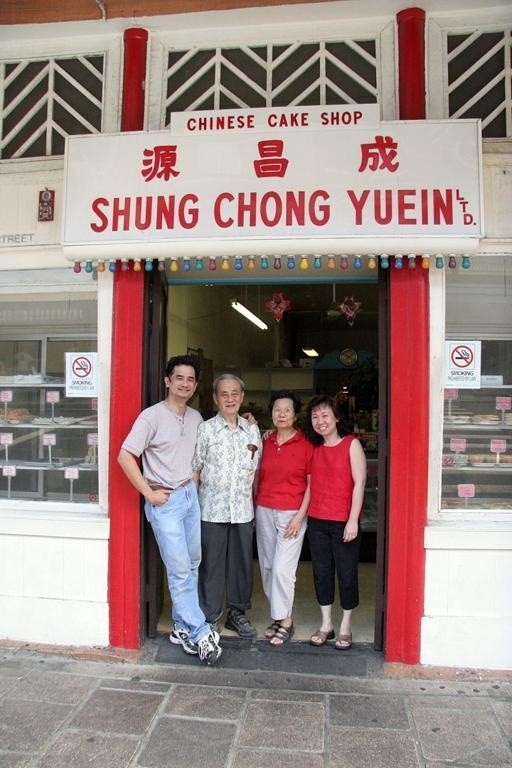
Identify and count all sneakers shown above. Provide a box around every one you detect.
[204,619,222,635]
[223,607,258,637]
[196,629,223,667]
[169,621,199,655]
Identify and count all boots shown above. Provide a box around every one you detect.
[263,619,283,640]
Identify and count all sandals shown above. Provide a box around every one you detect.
[268,620,296,647]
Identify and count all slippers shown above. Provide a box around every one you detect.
[310,628,336,647]
[334,631,353,650]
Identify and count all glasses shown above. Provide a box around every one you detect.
[272,407,295,417]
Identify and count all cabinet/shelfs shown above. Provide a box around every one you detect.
[441,386,512,510]
[0,383,97,502]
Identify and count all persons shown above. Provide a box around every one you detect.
[261,394,367,649]
[117,355,258,665]
[191,373,264,637]
[250,391,309,646]
